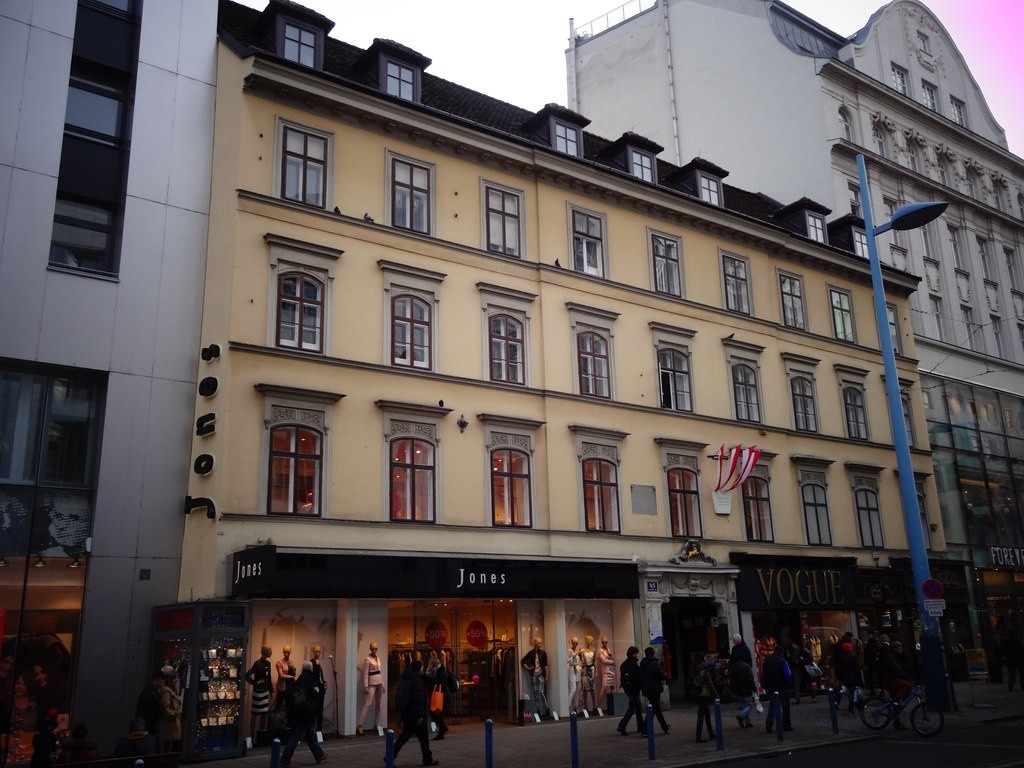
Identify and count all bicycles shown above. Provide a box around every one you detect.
[858,678,944,738]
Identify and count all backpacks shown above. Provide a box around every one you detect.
[289,679,319,706]
[687,668,707,690]
[396,685,412,714]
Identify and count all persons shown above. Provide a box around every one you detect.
[567,636,617,715]
[425,658,450,740]
[114,666,186,757]
[989,624,1024,691]
[356,642,386,735]
[692,652,721,743]
[832,630,915,730]
[760,629,778,667]
[521,638,551,719]
[640,647,671,737]
[278,661,328,768]
[29,720,100,768]
[616,647,646,735]
[724,634,759,729]
[245,644,328,744]
[761,643,796,734]
[793,644,817,705]
[384,660,440,766]
[0,643,65,762]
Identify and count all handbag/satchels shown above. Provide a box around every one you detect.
[449,671,459,693]
[803,662,823,682]
[430,684,443,715]
[852,685,865,704]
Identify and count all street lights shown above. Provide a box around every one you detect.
[852,152,958,712]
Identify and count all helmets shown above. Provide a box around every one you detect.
[160,665,177,688]
[890,641,902,647]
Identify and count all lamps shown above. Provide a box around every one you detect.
[0,557,8,567]
[67,558,82,568]
[32,557,47,567]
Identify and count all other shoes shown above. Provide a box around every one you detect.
[280,762,296,768]
[616,727,629,736]
[735,715,744,728]
[424,759,439,766]
[746,724,753,728]
[316,751,328,764]
[641,732,648,737]
[665,724,672,733]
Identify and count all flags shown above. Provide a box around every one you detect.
[715,446,761,492]
